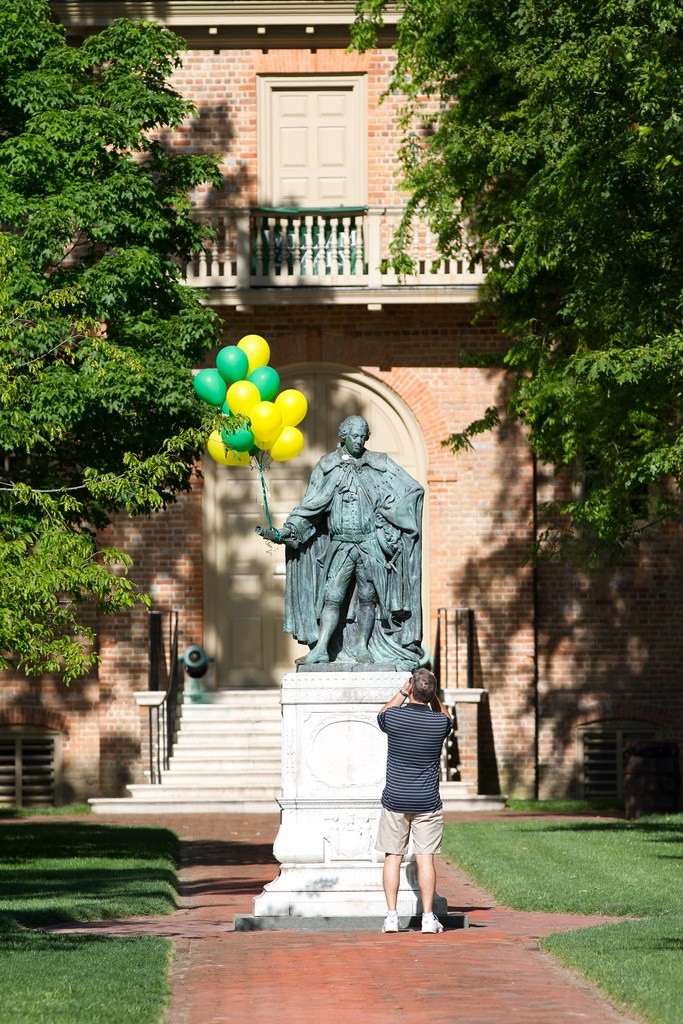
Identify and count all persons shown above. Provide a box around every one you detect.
[275,415,425,664]
[377,667,453,934]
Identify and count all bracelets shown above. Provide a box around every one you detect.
[399,690,408,697]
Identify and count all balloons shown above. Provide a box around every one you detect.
[226,380,260,418]
[207,428,251,467]
[194,367,227,407]
[217,346,248,384]
[249,366,280,399]
[221,401,230,416]
[250,401,281,443]
[275,389,307,427]
[238,334,270,377]
[221,420,253,452]
[253,435,270,450]
[271,428,303,462]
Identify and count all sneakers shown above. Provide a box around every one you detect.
[382,917,400,932]
[421,914,445,934]
